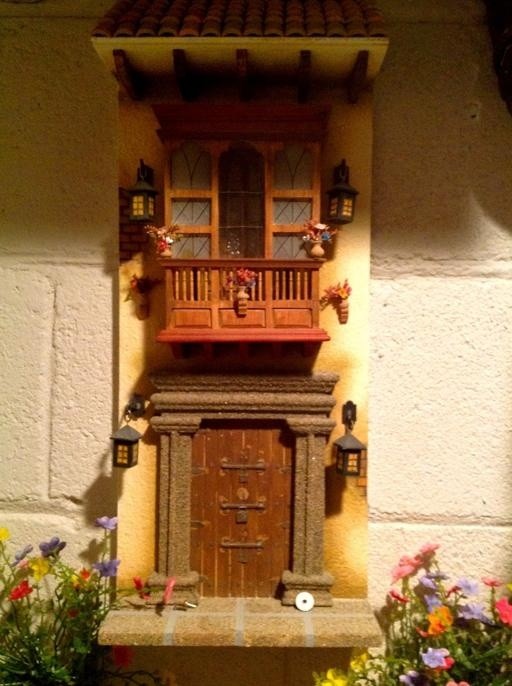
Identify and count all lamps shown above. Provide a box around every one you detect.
[129,156,160,222]
[325,158,359,224]
[332,401,366,477]
[109,402,143,468]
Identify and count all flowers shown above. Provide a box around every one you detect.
[316,276,353,308]
[301,217,338,242]
[126,272,165,293]
[142,221,184,252]
[225,267,259,290]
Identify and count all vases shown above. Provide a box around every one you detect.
[235,286,250,298]
[137,293,148,305]
[309,242,325,258]
[337,296,348,309]
[160,247,173,258]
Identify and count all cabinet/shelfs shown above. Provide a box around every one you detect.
[144,92,333,346]
